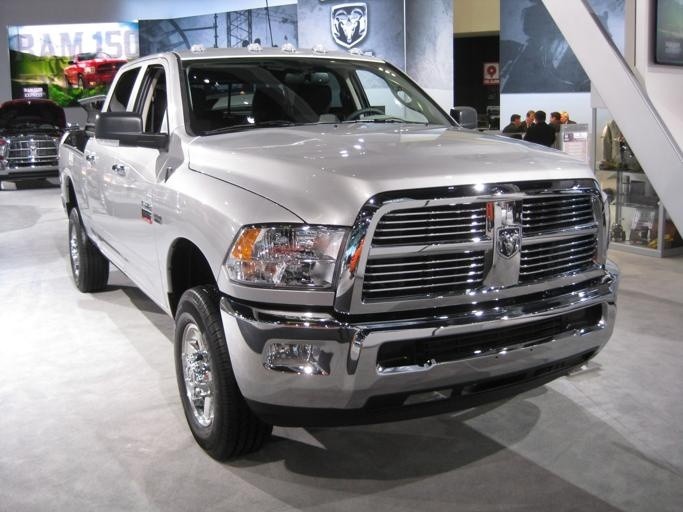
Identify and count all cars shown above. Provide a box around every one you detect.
[0,98,66,191]
[63,53,128,90]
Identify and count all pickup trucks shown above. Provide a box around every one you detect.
[57,51,619,462]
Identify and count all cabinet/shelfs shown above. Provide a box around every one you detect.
[589,81,683,259]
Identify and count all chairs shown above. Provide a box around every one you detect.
[251,86,289,125]
[295,81,332,123]
[190,87,216,133]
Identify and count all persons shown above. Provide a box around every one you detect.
[502,110,576,148]
